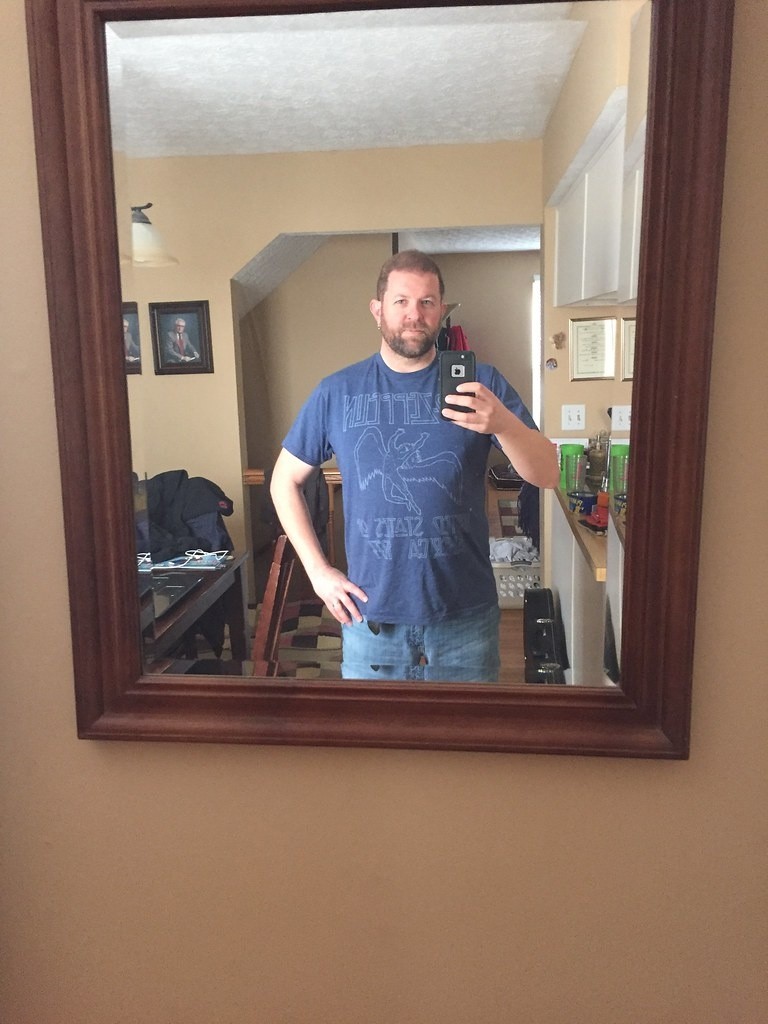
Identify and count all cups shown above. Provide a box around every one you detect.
[609,445,631,507]
[589,435,607,485]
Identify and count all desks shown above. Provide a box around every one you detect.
[243,467,344,572]
[154,550,251,663]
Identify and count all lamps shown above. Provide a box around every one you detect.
[131,202,179,267]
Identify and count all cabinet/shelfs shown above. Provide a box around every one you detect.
[555,116,644,310]
[487,483,523,539]
[549,491,625,688]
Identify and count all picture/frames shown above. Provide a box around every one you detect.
[148,298,215,376]
[121,301,142,375]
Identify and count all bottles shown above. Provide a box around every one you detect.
[596,493,609,524]
[559,443,587,495]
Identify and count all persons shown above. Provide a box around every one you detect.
[123,319,140,362]
[270,250,560,682]
[164,319,200,364]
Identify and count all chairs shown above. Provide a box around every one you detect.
[149,535,325,677]
[257,599,345,679]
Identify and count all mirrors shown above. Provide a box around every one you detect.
[25,0,736,759]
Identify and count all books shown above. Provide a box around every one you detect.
[138,556,228,575]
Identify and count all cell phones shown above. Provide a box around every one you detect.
[440,350,476,422]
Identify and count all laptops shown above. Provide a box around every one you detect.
[139,571,207,635]
